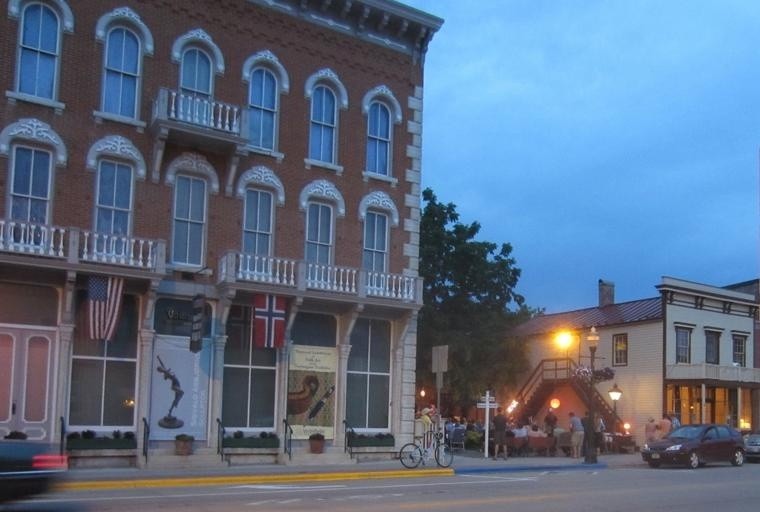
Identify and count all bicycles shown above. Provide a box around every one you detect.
[399,428,453,469]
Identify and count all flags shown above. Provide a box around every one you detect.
[253,294,287,350]
[87,275,125,341]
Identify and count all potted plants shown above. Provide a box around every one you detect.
[175,435,195,455]
[309,433,325,454]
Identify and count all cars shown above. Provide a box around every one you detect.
[744,433,760,463]
[0,438,70,506]
[640,424,747,470]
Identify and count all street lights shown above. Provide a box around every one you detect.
[585,325,600,464]
[609,384,623,454]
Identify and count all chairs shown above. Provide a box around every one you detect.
[505,435,555,458]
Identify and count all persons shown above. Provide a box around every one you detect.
[418,408,435,430]
[568,411,606,458]
[643,410,682,440]
[491,406,559,460]
[443,406,484,452]
